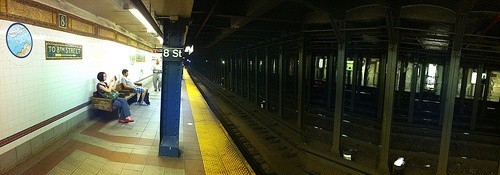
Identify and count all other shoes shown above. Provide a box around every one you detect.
[140,101,148,106]
[118,118,134,123]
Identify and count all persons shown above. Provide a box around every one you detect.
[152,58,162,91]
[121,69,149,105]
[96,72,134,123]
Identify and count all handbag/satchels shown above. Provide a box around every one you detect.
[138,92,150,105]
[102,89,119,102]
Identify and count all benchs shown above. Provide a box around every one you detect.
[92,83,143,122]
